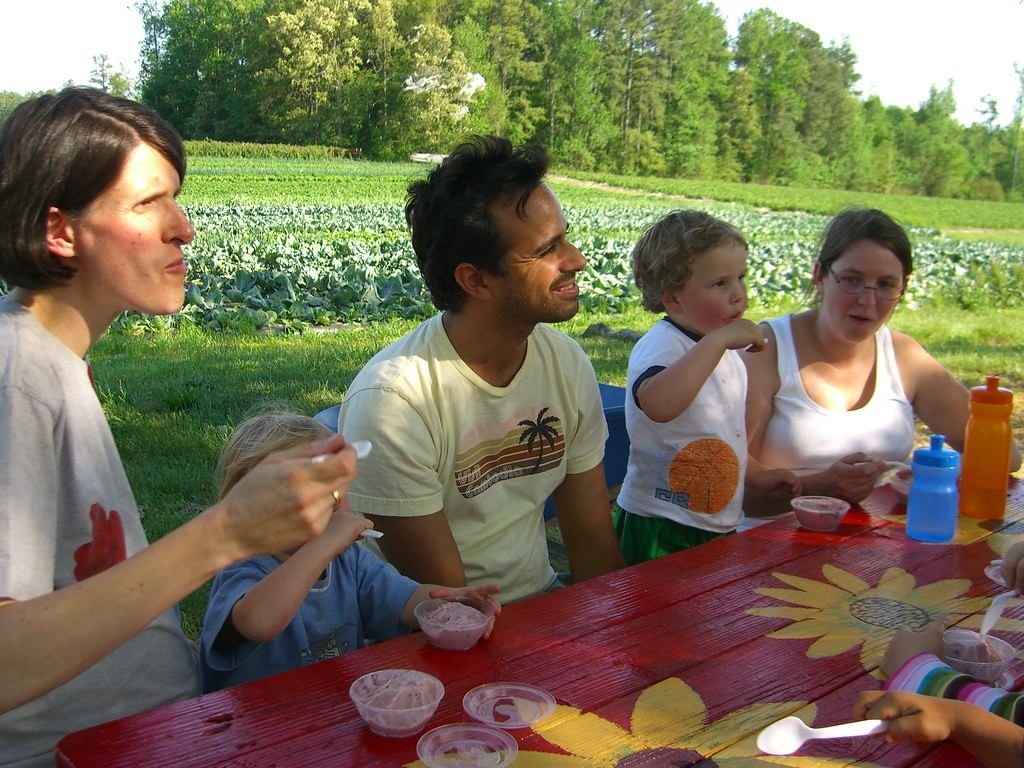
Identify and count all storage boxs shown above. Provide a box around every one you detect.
[315,382,630,519]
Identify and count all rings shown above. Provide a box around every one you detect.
[331,490,340,505]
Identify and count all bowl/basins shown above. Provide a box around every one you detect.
[891,478,910,504]
[349,668,445,738]
[944,634,1015,687]
[791,496,850,532]
[414,596,494,650]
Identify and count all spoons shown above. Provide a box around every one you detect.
[744,338,769,351]
[757,716,891,756]
[855,461,910,470]
[312,441,371,463]
[981,591,1017,635]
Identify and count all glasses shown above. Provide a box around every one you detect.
[827,262,906,300]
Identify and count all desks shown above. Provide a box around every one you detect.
[54,475,1024,768]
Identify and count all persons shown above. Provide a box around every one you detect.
[0,87,356,767]
[853,539,1024,768]
[202,412,497,690]
[332,139,626,600]
[614,212,798,566]
[738,209,1023,532]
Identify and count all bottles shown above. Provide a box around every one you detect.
[960,374,1013,518]
[906,437,960,543]
